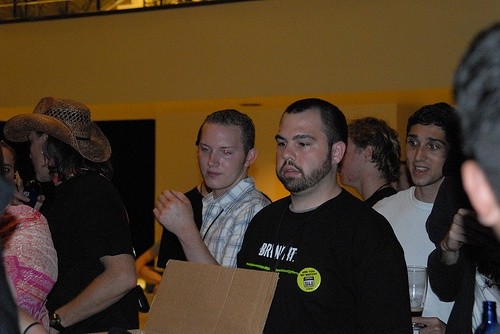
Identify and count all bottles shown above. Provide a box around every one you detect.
[474,300,500,334]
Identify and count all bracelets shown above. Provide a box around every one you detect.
[47,310,66,332]
[23,321,42,334]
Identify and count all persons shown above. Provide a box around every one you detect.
[0,96,137,334]
[237,97,414,334]
[153,107,274,269]
[337,20,500,334]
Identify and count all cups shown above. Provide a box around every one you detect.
[406,266,428,330]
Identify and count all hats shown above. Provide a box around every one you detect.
[4,96,111,162]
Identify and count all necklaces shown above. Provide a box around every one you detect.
[197,180,206,196]
[373,182,390,194]
